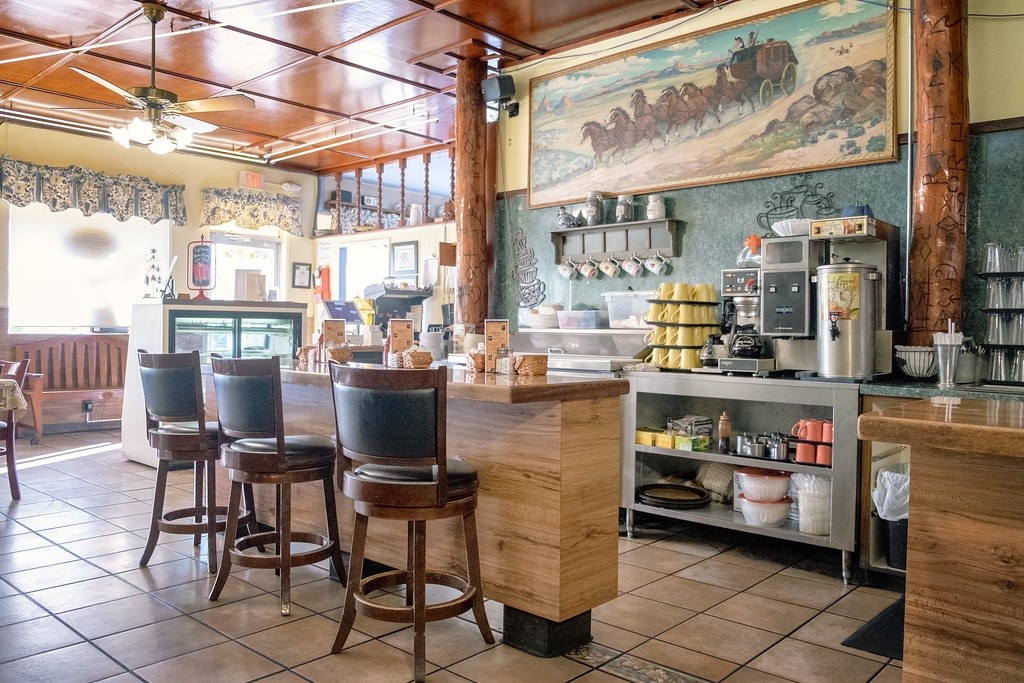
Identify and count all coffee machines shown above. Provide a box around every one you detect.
[690,267,776,377]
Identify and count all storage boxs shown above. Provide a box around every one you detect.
[600,289,657,328]
[558,309,608,330]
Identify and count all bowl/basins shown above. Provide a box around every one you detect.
[738,469,791,502]
[737,493,792,528]
[772,218,815,237]
[419,331,445,361]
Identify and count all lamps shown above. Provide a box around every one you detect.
[111,119,178,152]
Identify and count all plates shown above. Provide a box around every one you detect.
[243,350,267,352]
[634,483,711,509]
[525,313,559,328]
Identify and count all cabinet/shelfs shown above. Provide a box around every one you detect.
[621,370,862,589]
[123,300,304,470]
[976,269,1024,387]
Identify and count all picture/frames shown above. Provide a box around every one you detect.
[292,262,311,289]
[525,1,895,209]
[393,275,417,289]
[389,240,419,275]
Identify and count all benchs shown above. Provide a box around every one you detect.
[15,334,128,445]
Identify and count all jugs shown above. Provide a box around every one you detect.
[736,234,761,268]
[728,322,765,356]
[697,334,727,365]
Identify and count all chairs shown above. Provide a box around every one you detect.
[0,358,31,499]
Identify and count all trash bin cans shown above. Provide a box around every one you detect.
[881,461,912,570]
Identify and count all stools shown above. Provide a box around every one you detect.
[138,345,266,567]
[207,351,348,617]
[328,360,495,680]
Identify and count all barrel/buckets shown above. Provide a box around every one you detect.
[811,257,882,380]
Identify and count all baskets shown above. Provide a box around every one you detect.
[514,355,548,377]
[465,354,486,373]
[324,348,353,363]
[298,345,316,361]
[389,352,404,368]
[403,351,432,369]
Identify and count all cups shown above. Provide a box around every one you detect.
[796,490,830,536]
[557,250,668,279]
[736,417,832,465]
[933,344,960,387]
[955,353,977,384]
[643,282,720,371]
[982,241,1024,386]
[974,357,982,382]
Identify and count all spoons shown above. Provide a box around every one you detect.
[960,339,986,354]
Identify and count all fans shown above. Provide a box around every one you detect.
[70,2,255,134]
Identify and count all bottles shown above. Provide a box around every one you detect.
[477,342,516,375]
[718,411,731,453]
[586,190,666,226]
